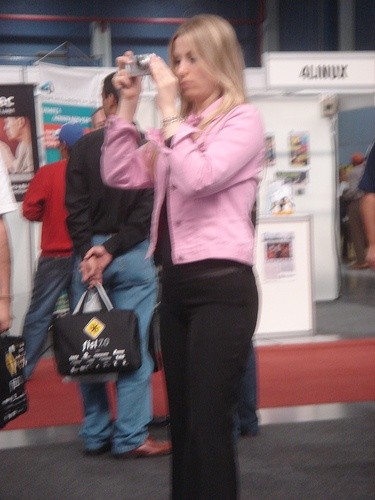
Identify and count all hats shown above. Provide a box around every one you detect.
[351,152,366,162]
[54,121,84,148]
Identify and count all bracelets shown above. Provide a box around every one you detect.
[0,296,10,301]
[163,114,181,126]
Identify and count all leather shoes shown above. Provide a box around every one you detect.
[113,435,172,458]
[86,445,107,458]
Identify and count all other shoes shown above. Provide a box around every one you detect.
[348,259,371,269]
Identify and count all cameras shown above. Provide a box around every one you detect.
[128,52,156,77]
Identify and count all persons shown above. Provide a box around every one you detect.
[233,345,261,439]
[100,13,265,500]
[92,106,107,129]
[338,144,375,269]
[0,154,18,333]
[18,123,87,382]
[0,115,34,174]
[63,70,173,458]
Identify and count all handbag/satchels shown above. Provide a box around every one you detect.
[0,333,29,431]
[48,279,143,377]
